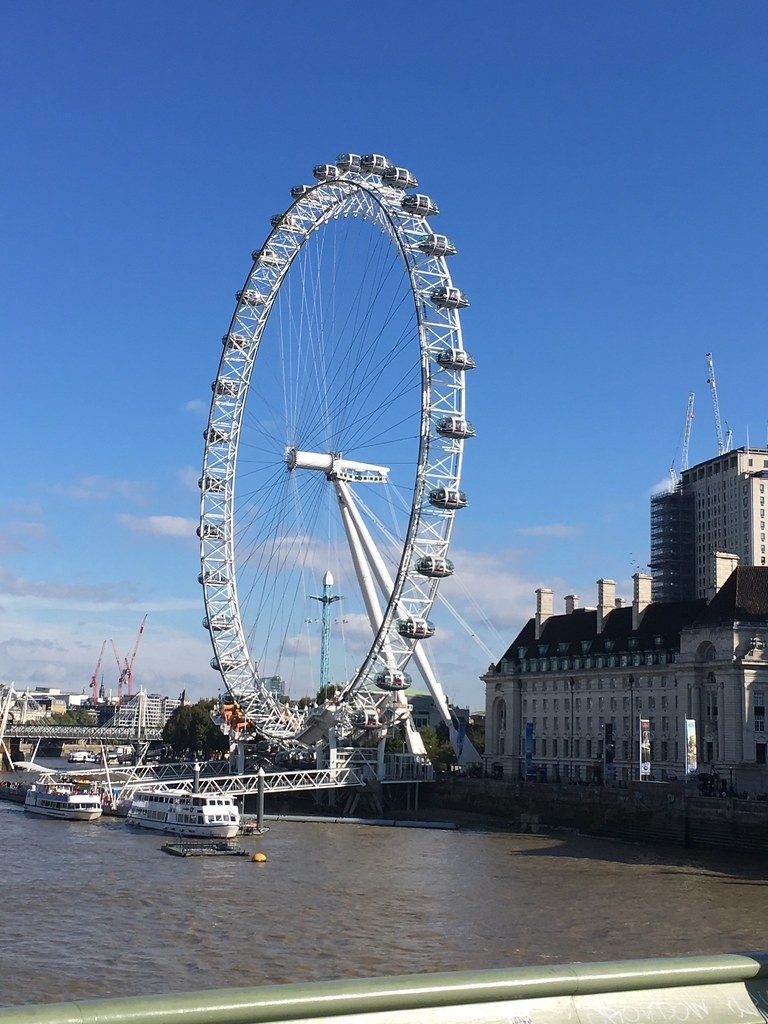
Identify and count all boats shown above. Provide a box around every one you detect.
[67,751,95,763]
[24,781,103,820]
[124,789,240,839]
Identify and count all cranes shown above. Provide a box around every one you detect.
[89,613,149,704]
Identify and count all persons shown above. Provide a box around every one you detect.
[704,787,758,800]
[0,779,78,798]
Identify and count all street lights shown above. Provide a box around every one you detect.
[569,676,574,785]
[516,677,524,782]
[728,766,733,793]
[628,673,634,781]
